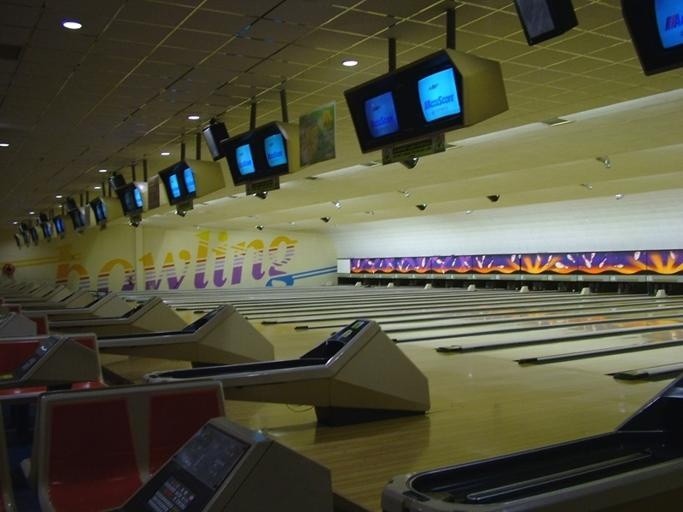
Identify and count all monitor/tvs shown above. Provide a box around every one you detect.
[231,136,273,180]
[181,164,197,198]
[415,59,464,141]
[359,83,436,148]
[71,211,85,229]
[262,131,288,175]
[165,169,184,203]
[620,0,683,76]
[94,201,107,222]
[55,216,65,233]
[122,186,143,213]
[42,222,53,237]
[15,228,38,247]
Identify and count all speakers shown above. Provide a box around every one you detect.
[512,0,579,47]
[67,199,77,209]
[200,122,231,161]
[19,223,28,233]
[108,175,125,193]
[38,214,48,225]
